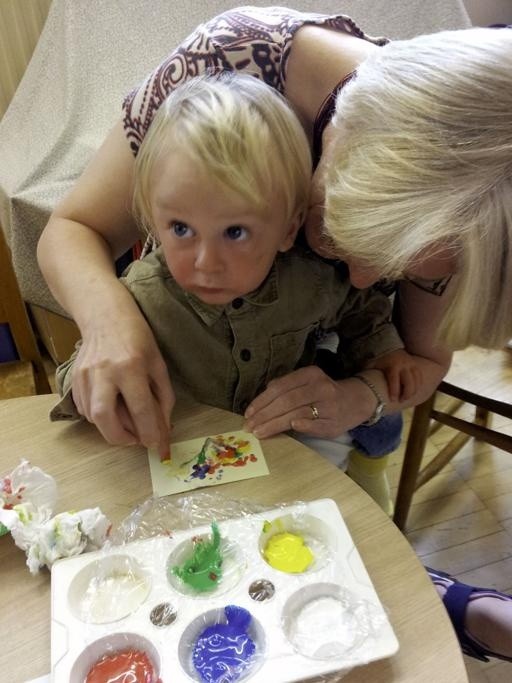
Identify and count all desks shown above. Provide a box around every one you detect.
[3,388,473,683]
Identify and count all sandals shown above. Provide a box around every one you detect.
[426,567,510,662]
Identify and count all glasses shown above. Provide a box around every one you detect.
[399,274,456,297]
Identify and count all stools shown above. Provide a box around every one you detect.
[394,346,511,532]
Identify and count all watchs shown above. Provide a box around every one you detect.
[353,372,387,428]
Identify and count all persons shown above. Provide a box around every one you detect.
[36,2,510,452]
[47,73,427,470]
[418,556,511,662]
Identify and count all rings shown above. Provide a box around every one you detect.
[306,401,322,421]
